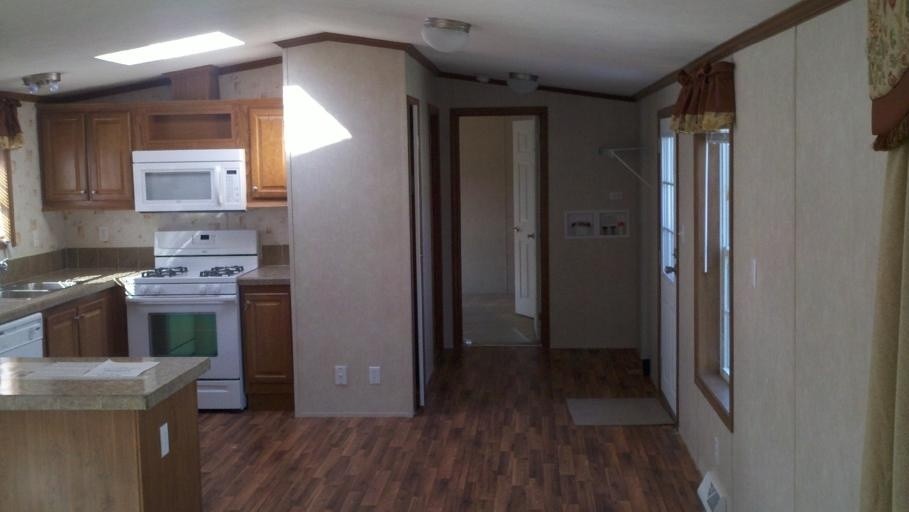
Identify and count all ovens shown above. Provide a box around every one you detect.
[125,296,248,409]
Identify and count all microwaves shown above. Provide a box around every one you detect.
[131,149,247,213]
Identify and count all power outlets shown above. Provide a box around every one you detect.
[369,367,381,385]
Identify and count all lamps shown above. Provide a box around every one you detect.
[22,72,62,96]
[507,73,539,94]
[421,17,472,53]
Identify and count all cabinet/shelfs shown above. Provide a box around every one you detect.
[238,285,294,410]
[37,101,135,210]
[244,98,287,207]
[43,287,114,358]
[133,99,244,151]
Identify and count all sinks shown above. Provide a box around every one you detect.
[1,279,75,290]
[0,290,49,299]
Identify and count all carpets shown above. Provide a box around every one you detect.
[566,397,674,427]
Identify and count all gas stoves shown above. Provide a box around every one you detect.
[124,265,262,296]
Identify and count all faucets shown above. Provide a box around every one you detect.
[0,256,10,273]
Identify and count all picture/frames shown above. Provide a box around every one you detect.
[563,210,597,240]
[596,210,630,238]
